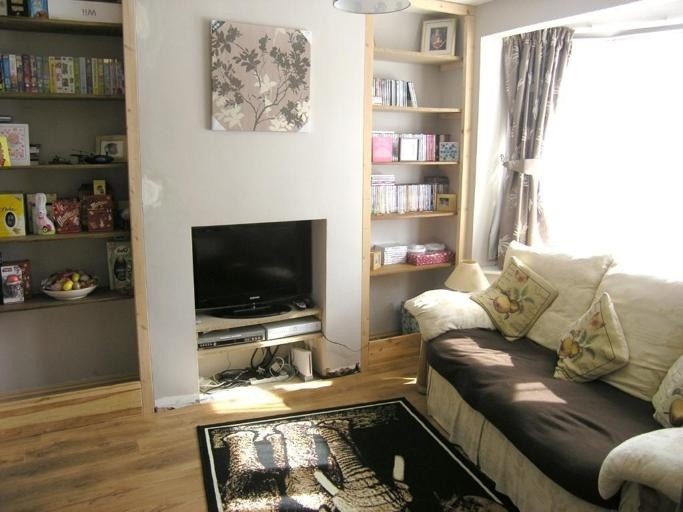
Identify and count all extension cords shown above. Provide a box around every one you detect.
[249,371,288,384]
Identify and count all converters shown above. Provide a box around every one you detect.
[271,361,280,373]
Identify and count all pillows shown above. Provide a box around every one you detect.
[653,354,683,426]
[505,240,614,352]
[553,292,631,383]
[589,271,682,402]
[471,257,558,344]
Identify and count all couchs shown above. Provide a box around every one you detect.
[400,238,683,511]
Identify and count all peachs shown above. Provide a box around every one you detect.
[669,397,683,427]
[488,295,520,313]
[559,337,580,358]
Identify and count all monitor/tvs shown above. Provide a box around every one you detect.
[191,220,312,319]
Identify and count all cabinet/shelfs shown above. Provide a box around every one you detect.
[0,1,144,398]
[194,299,323,354]
[363,0,480,374]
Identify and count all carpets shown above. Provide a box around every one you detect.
[194,397,521,512]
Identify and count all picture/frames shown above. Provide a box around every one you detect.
[436,193,456,211]
[96,134,127,162]
[419,18,458,58]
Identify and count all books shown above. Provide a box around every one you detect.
[1,54,125,96]
[372,17,456,269]
[2,0,49,19]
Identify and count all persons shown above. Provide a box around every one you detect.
[7,134,23,155]
[107,143,118,155]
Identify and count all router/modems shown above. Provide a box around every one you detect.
[291,349,313,376]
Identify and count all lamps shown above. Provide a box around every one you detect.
[443,261,492,294]
[332,1,413,15]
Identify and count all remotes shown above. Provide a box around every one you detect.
[291,299,306,310]
